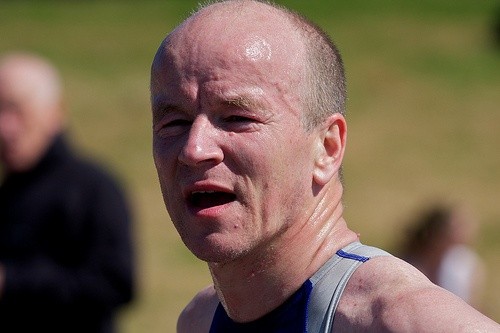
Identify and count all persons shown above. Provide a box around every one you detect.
[147,1,500,333]
[0,53,140,333]
[397,198,477,310]
